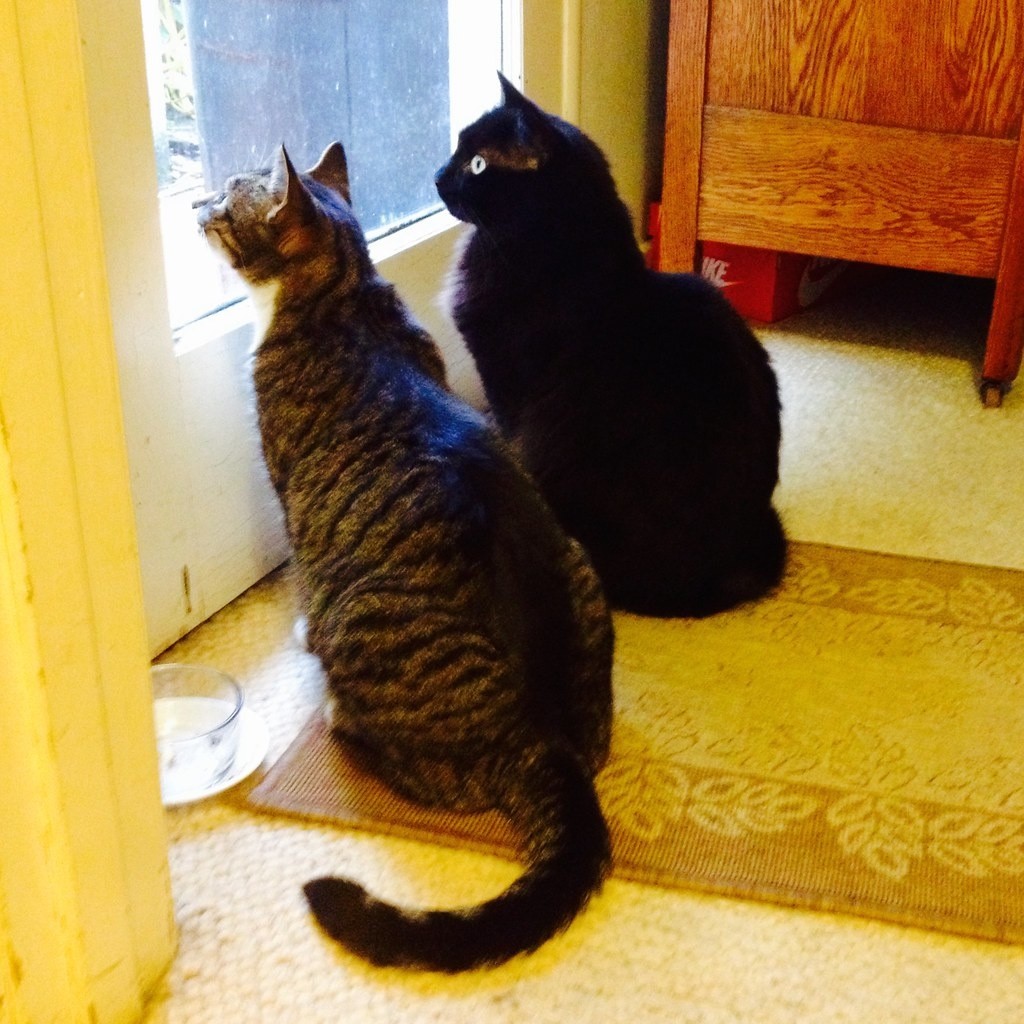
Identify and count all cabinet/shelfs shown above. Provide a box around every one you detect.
[649,0,1024,411]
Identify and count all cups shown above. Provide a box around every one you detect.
[149,663,243,797]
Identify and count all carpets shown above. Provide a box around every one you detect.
[247,541,1024,952]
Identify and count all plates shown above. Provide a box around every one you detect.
[154,696,270,803]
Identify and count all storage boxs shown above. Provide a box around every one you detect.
[647,204,844,327]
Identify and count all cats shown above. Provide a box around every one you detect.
[192,69,788,974]
